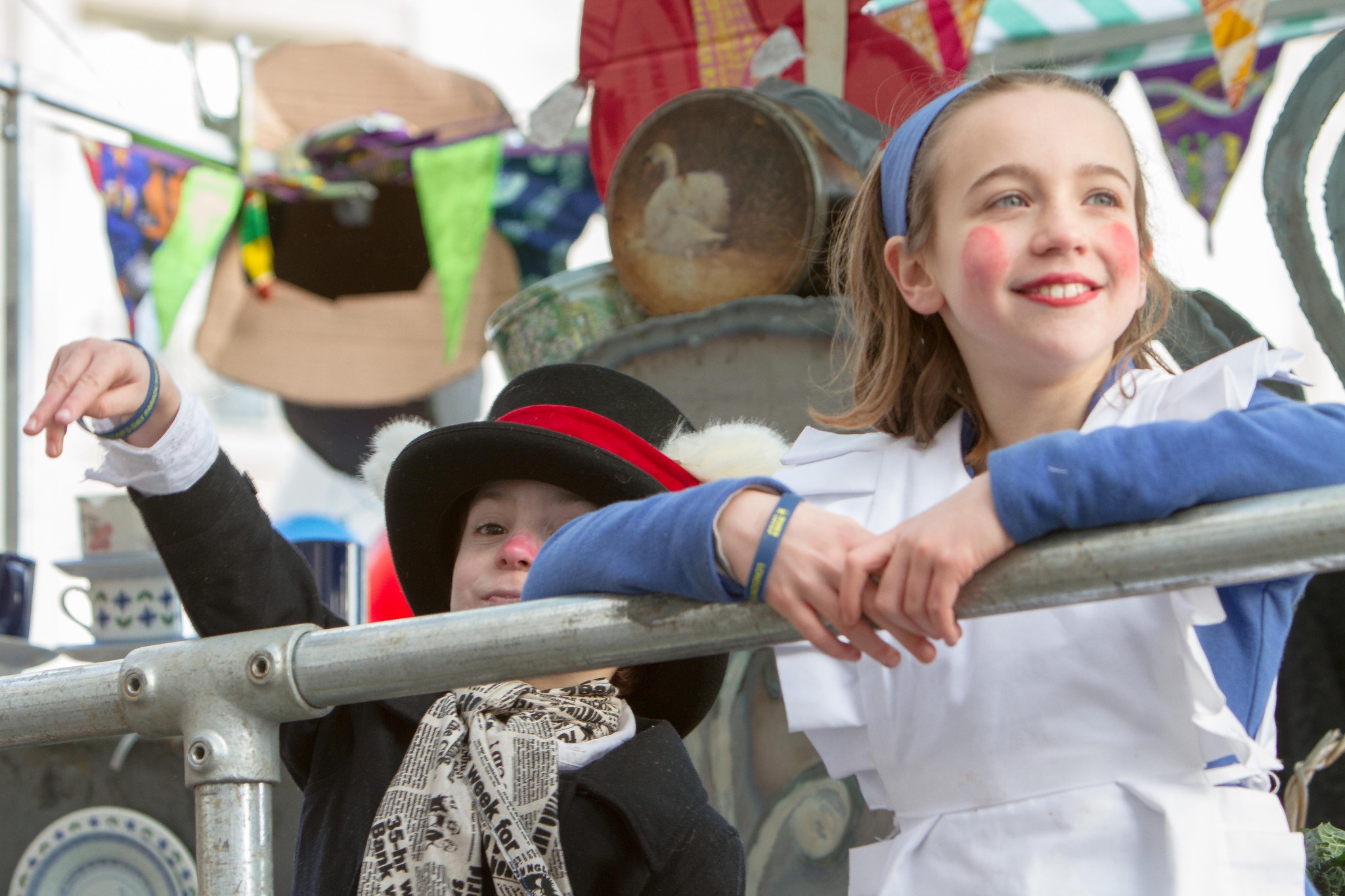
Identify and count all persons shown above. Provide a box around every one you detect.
[24,339,745,896]
[525,70,1345,896]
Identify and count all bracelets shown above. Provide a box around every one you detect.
[76,338,159,440]
[744,493,804,604]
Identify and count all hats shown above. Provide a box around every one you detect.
[383,362,732,740]
